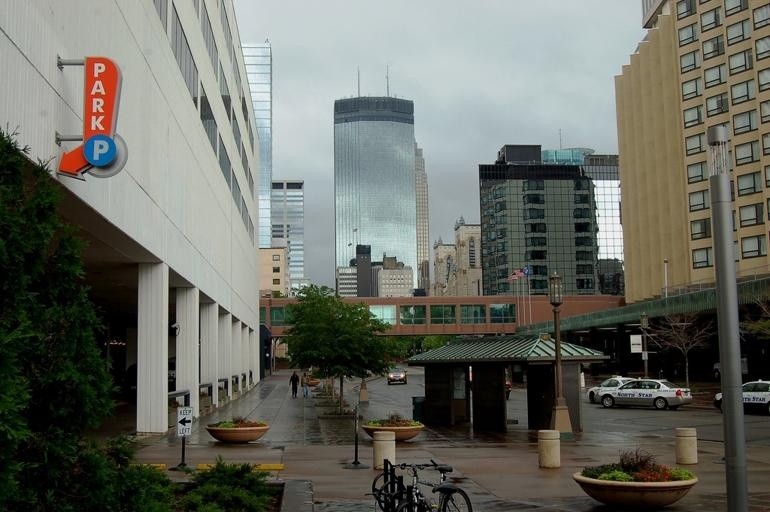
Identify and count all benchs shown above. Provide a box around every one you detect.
[196,461,284,473]
[132,463,166,471]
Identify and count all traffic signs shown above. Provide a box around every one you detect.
[177,407,192,437]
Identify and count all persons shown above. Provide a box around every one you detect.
[301,372,310,397]
[288,370,300,399]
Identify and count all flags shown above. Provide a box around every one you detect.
[506,266,528,280]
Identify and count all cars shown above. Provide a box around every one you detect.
[713,378,770,415]
[587,375,638,404]
[386,366,407,385]
[596,376,693,409]
[469,366,512,399]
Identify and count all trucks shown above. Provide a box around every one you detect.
[713,334,769,380]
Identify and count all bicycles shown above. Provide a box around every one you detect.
[372,459,473,512]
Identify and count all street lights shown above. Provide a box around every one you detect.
[663,257,670,299]
[640,311,649,380]
[547,269,574,439]
